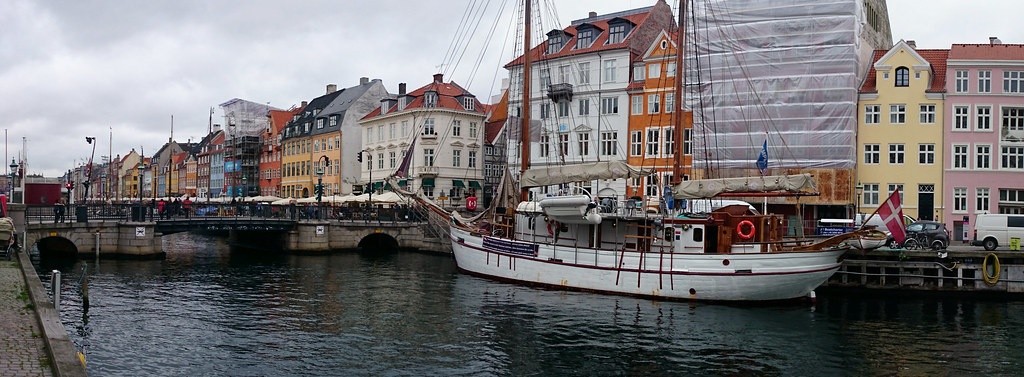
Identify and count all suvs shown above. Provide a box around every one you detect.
[905,221,950,249]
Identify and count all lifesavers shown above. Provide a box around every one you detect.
[547,220,561,235]
[736,220,756,239]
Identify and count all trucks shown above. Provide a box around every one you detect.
[854,213,917,231]
[687,199,761,216]
[971,212,1024,251]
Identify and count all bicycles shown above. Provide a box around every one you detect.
[904,231,944,250]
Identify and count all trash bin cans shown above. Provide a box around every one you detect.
[1010,237,1021,251]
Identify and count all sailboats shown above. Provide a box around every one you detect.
[383,0,907,301]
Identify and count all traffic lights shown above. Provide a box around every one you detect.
[315,185,320,195]
[70,180,75,190]
[372,183,376,193]
[66,184,71,189]
[325,157,329,166]
[321,185,325,194]
[357,152,362,162]
[366,183,371,193]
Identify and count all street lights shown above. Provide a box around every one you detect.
[136,162,146,221]
[484,143,494,198]
[317,168,324,205]
[9,157,18,203]
[856,180,863,214]
[242,174,246,202]
[101,174,106,215]
[154,163,157,208]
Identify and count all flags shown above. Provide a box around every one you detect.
[878,191,907,244]
[756,139,768,175]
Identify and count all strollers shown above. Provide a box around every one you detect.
[0,218,15,261]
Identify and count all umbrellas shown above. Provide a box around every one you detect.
[78,188,416,203]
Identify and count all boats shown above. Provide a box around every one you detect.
[848,229,887,251]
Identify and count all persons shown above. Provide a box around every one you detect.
[393,202,401,223]
[290,200,296,219]
[230,197,256,216]
[300,202,318,220]
[143,198,156,220]
[408,203,421,221]
[158,196,192,220]
[54,198,66,223]
